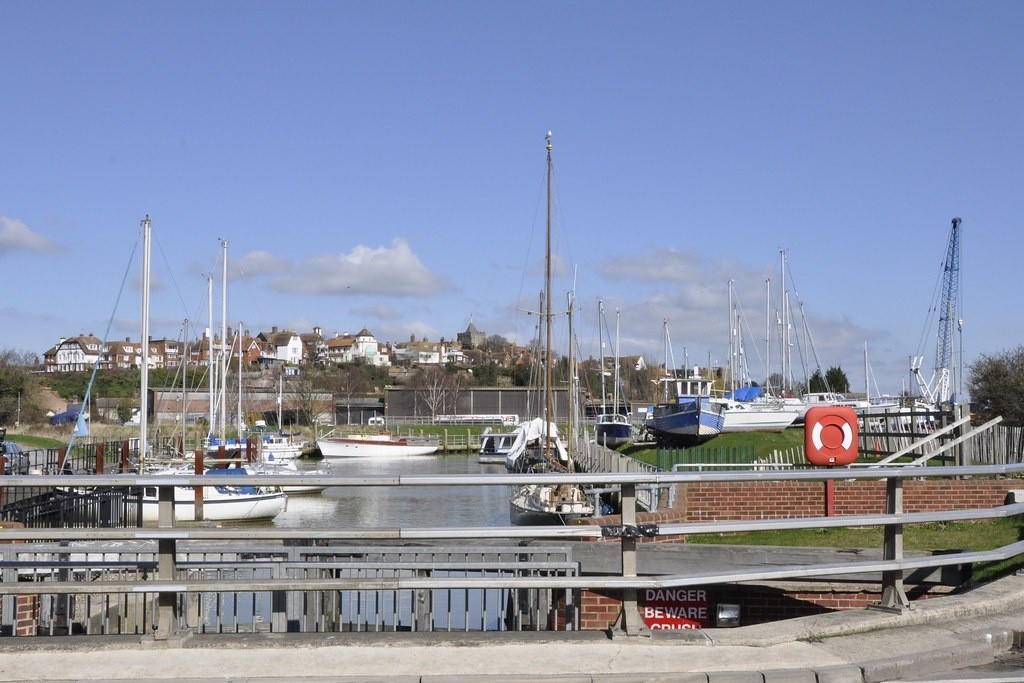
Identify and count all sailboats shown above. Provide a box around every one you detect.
[475,130,594,527]
[135,235,330,492]
[645,248,936,443]
[593,309,633,449]
[33,213,288,521]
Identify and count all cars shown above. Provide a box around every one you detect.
[2,441,30,475]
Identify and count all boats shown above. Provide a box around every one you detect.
[316,428,440,457]
